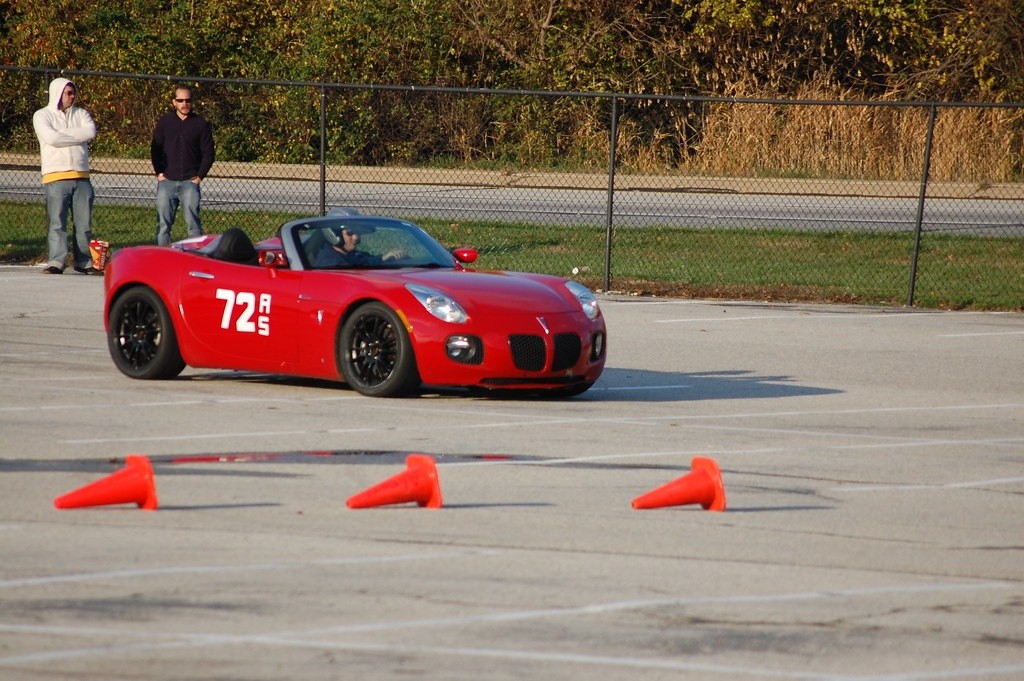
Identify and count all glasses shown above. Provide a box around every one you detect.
[63,91,76,96]
[174,98,192,103]
[344,227,360,236]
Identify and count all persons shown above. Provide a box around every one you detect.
[151,84,217,246]
[33,78,104,275]
[315,223,405,267]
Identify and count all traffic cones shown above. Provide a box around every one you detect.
[53,454,159,511]
[629,457,728,514]
[346,452,442,508]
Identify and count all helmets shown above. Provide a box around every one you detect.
[325,206,358,246]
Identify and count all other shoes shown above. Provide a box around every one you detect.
[74,266,105,275]
[43,266,63,275]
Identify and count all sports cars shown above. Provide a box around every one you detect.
[100,212,608,399]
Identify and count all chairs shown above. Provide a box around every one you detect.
[302,229,325,266]
[212,227,259,266]
